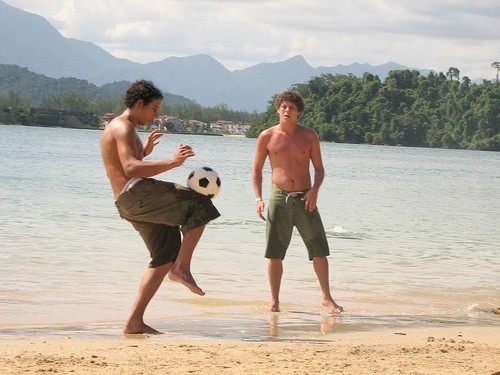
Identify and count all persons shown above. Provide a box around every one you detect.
[252,90,344,315]
[100,79,221,335]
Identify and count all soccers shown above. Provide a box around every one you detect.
[187,166,221,198]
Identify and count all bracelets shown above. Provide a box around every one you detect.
[255,198,262,202]
